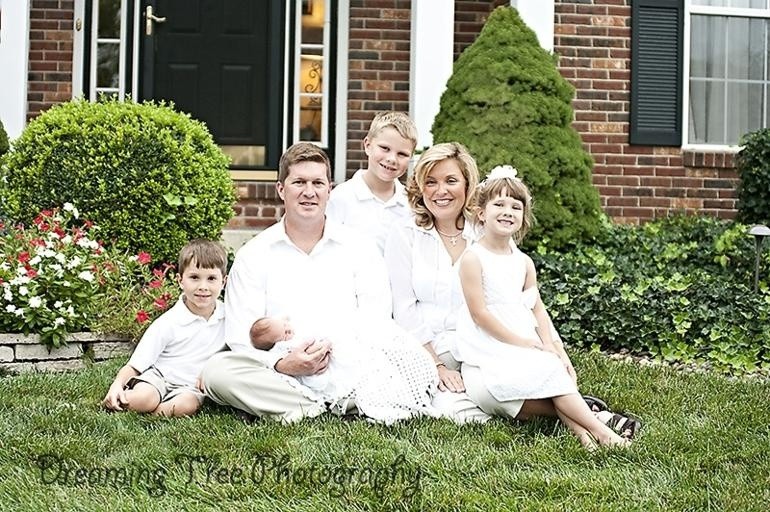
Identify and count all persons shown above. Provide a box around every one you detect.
[101,237,226,420]
[200,141,392,424]
[451,164,634,457]
[325,109,413,221]
[249,314,333,374]
[390,141,642,442]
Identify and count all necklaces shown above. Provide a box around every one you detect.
[435,224,466,247]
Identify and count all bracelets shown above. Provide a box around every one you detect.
[435,361,446,368]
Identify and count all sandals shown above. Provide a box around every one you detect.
[583,393,642,440]
